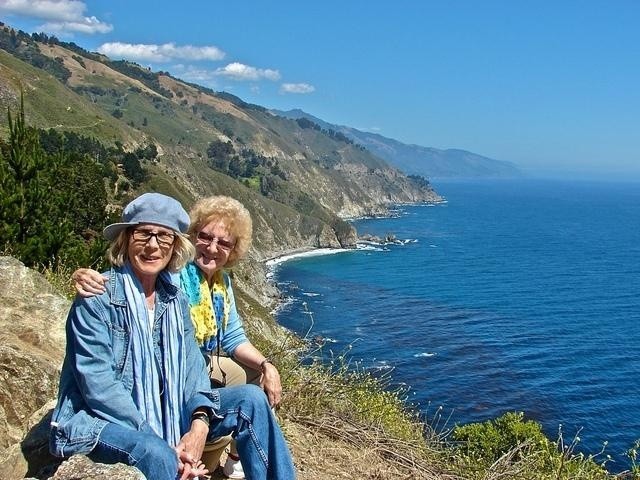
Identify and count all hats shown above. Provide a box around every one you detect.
[103,192,190,242]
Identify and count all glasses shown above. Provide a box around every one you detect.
[132,228,176,245]
[193,228,234,251]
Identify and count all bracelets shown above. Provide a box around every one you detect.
[258,358,274,372]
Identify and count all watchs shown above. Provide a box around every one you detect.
[191,412,209,427]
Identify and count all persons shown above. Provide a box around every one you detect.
[70,194,284,479]
[46,192,297,479]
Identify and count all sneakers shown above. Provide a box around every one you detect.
[223,452,245,479]
